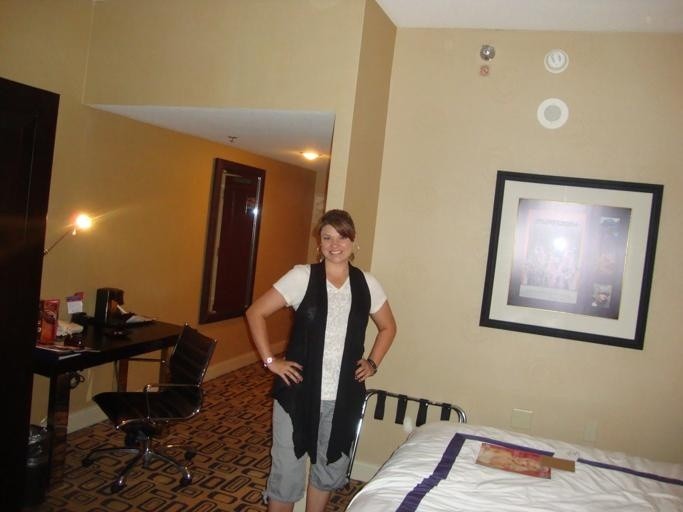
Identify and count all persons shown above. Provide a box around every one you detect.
[244,209,398,512]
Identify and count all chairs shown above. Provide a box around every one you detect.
[82,321,218,491]
[350,390,467,486]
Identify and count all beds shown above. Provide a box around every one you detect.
[345,420,683,512]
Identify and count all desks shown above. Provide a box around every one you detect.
[35,316,187,472]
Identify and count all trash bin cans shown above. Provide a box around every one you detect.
[29,424,48,504]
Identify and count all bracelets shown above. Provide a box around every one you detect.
[367,359,377,373]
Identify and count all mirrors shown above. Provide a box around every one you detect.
[198,156,266,323]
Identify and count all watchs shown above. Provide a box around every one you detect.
[264,356,277,368]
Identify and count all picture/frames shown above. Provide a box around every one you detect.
[478,170,665,352]
